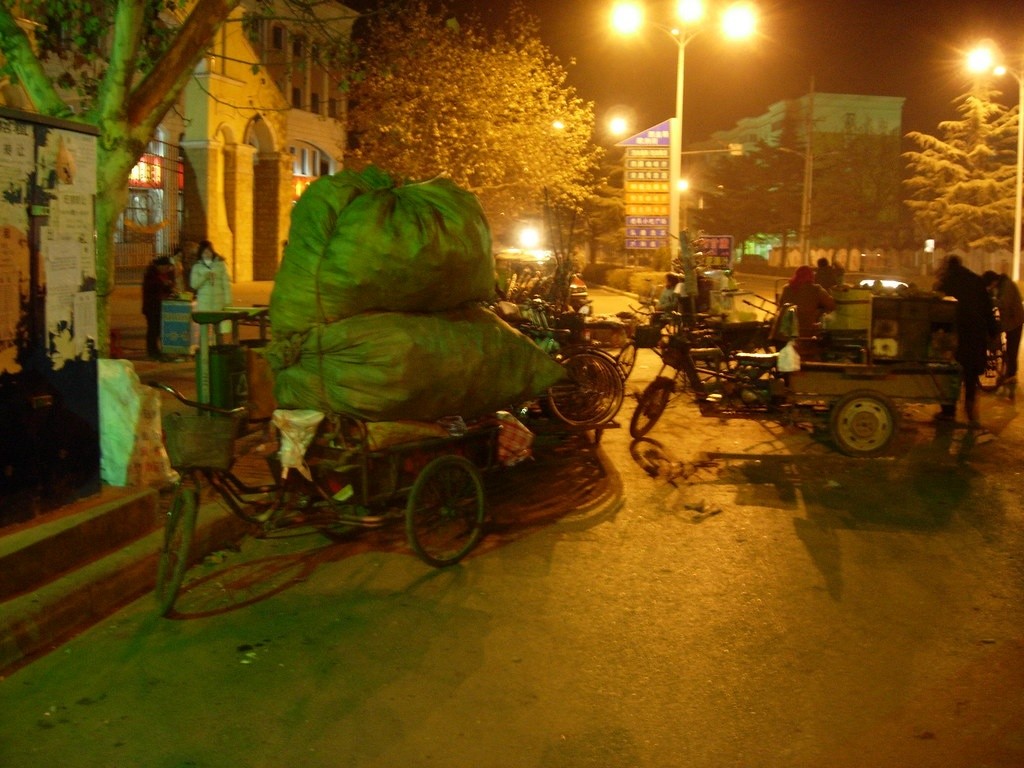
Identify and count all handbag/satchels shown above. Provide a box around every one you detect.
[219,319,232,333]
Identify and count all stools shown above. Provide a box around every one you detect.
[735,353,779,384]
[273,408,324,468]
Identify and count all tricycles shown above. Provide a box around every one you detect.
[130,373,504,620]
[629,294,963,457]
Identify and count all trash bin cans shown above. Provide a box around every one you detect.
[190,303,277,423]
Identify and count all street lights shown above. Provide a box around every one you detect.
[965,45,1024,288]
[602,1,763,291]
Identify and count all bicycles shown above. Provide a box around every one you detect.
[978,306,1011,393]
[484,293,627,430]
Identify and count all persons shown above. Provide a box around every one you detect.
[276,239,289,265]
[652,257,846,386]
[142,241,230,362]
[930,255,994,427]
[978,270,1024,383]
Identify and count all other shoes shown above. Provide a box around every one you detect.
[935,411,955,419]
